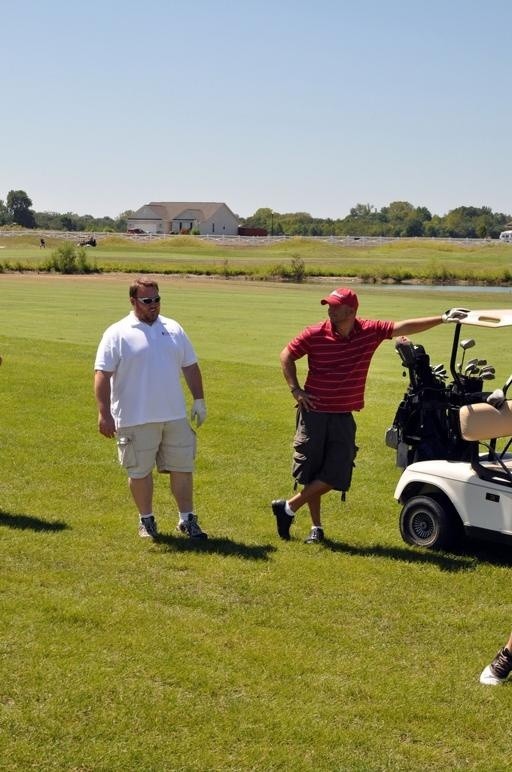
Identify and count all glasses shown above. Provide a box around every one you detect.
[136,296,161,305]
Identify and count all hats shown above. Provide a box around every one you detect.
[320,287,359,308]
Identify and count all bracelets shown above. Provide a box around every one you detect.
[291,386,299,393]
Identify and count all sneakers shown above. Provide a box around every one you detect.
[175,513,208,539]
[137,516,160,537]
[271,499,294,540]
[479,647,512,687]
[304,528,324,544]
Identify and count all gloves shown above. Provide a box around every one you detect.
[442,307,471,323]
[191,398,207,428]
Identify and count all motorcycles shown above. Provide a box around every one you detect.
[393,302,512,551]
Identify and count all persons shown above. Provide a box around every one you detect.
[270,287,472,545]
[477,626,511,689]
[92,279,210,542]
